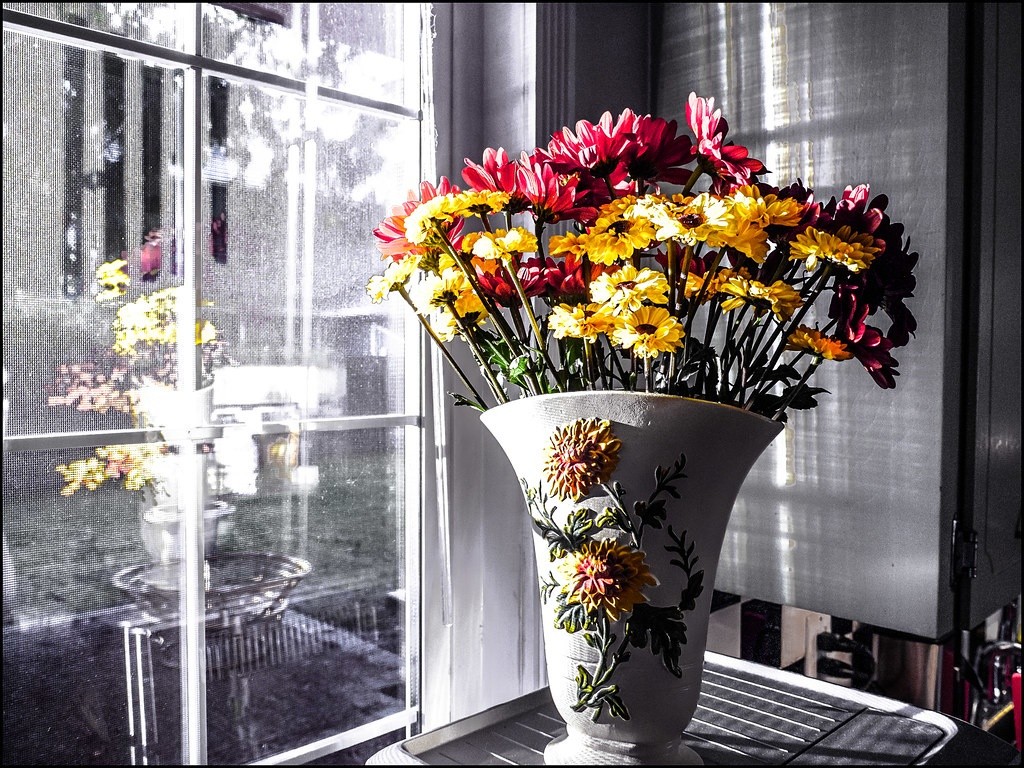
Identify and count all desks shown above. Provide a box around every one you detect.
[365,650,964,765]
[0,562,406,768]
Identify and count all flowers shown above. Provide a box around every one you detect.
[366,90,920,422]
[49,255,222,497]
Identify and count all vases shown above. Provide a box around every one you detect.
[479,391,785,765]
[145,384,236,562]
